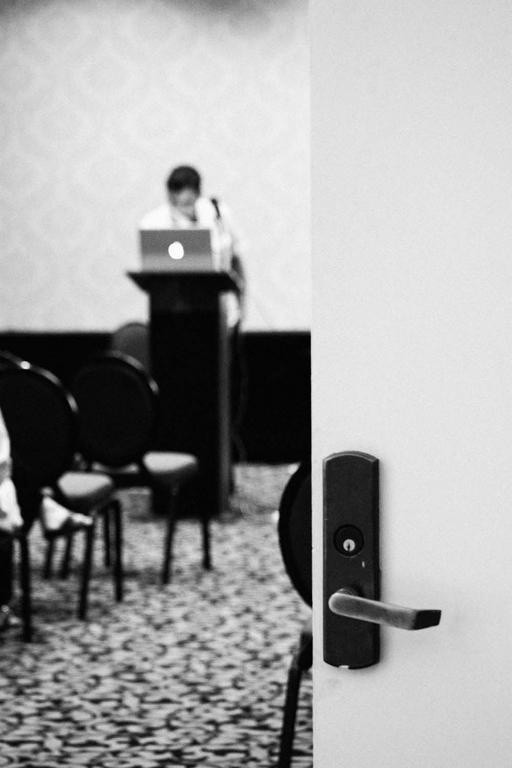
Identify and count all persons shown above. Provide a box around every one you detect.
[138,166,242,335]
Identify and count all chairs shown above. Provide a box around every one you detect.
[277,459,313,768]
[0,349,215,621]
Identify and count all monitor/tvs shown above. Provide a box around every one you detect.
[139,228,213,271]
